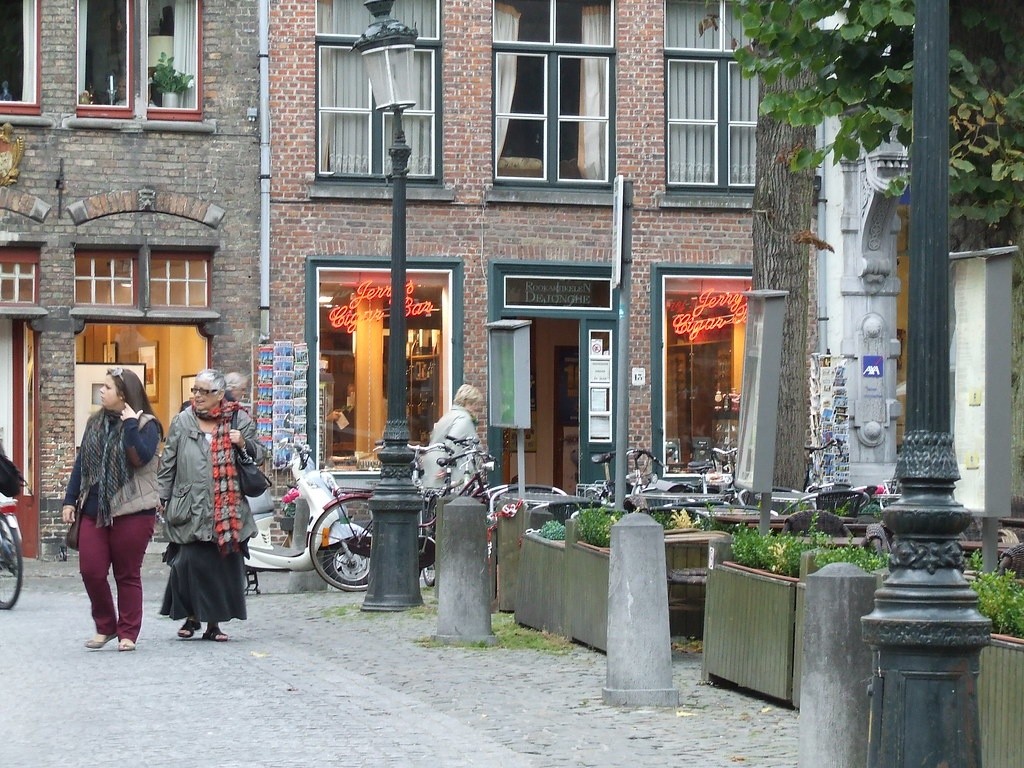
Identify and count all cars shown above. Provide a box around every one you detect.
[237,436,893,578]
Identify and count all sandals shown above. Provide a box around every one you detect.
[202,627,228,642]
[178,620,201,638]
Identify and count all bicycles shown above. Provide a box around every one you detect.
[0,493,25,610]
[306,435,498,592]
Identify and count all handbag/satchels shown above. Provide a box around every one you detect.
[65,499,83,552]
[232,411,272,497]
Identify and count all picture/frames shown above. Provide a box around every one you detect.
[135,338,160,404]
[103,341,116,362]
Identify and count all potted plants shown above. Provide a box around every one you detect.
[966,546,1024,768]
[701,524,888,707]
[520,504,746,653]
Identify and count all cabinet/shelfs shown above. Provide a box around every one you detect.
[409,333,442,440]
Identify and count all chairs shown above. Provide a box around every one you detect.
[491,481,882,612]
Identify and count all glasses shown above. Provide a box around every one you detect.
[110,366,124,382]
[190,387,219,396]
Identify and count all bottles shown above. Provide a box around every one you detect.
[723,388,731,412]
[714,383,723,411]
[730,388,739,412]
[80,93,89,104]
[0,81,12,101]
[421,399,427,415]
[422,364,428,378]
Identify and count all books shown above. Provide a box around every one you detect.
[255,340,310,470]
[406,328,441,380]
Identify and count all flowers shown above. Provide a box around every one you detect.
[283,488,301,518]
[148,53,195,97]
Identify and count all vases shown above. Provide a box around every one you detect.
[162,93,179,107]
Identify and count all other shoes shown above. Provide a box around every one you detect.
[84,631,118,648]
[118,639,135,651]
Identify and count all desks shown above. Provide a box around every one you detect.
[713,513,856,524]
[641,489,721,508]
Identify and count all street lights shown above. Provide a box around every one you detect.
[344,1,423,612]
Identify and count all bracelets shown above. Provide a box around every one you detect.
[243,442,246,449]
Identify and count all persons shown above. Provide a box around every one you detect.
[422,384,483,534]
[63,368,163,651]
[325,409,342,422]
[158,369,265,641]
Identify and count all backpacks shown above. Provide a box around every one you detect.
[0,442,23,497]
